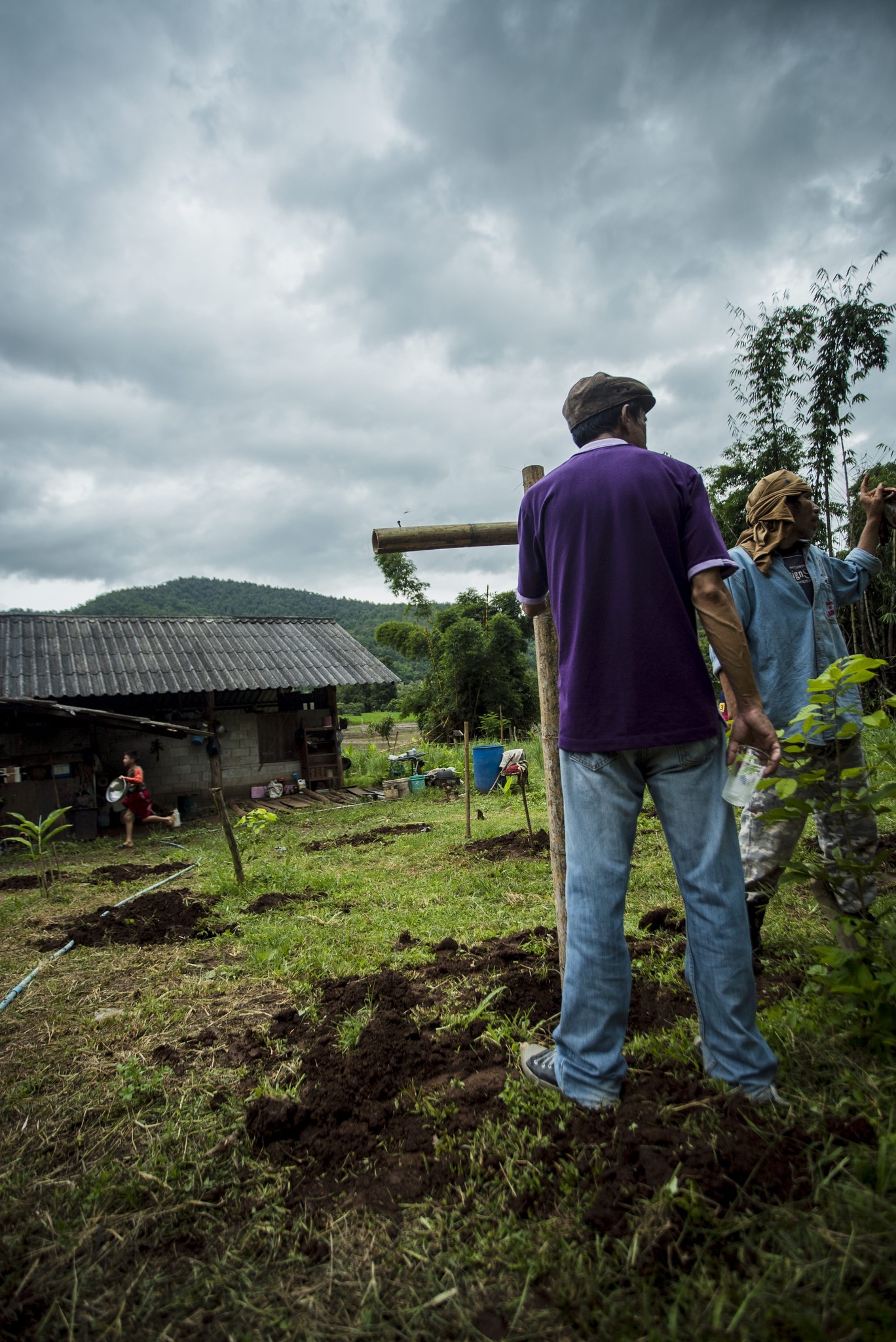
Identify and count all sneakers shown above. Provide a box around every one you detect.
[519,1041,617,1111]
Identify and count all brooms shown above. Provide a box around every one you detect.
[47,769,75,845]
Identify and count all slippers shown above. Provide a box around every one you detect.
[115,844,132,849]
[168,816,174,831]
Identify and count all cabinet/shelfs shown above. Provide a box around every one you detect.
[305,726,344,793]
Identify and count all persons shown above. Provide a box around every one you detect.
[115,749,175,849]
[709,466,889,974]
[520,370,791,1114]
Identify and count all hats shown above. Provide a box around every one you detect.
[563,372,655,433]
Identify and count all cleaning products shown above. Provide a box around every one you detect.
[297,778,308,795]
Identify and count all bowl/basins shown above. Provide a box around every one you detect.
[106,777,129,803]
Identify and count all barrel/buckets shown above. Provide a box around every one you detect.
[409,775,426,794]
[326,769,334,777]
[395,778,410,798]
[472,745,506,795]
[382,780,399,800]
[69,807,99,843]
[298,779,307,794]
